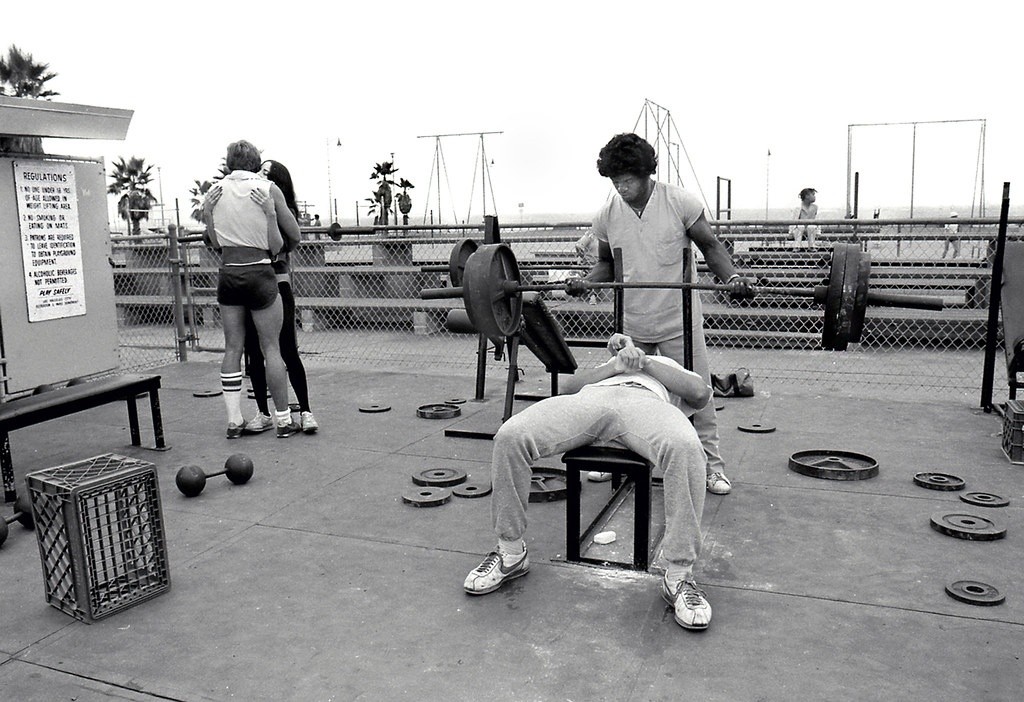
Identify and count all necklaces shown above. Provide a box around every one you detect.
[631,206,646,217]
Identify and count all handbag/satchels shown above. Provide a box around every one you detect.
[711,367,755,398]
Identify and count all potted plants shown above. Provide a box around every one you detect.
[363,190,394,225]
[394,178,414,214]
[368,161,399,209]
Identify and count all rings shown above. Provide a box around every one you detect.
[209,194,212,197]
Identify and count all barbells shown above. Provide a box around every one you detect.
[419,242,944,352]
[420,238,595,287]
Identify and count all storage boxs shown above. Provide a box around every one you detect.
[24,450,171,626]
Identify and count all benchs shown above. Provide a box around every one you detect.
[111,226,996,347]
[561,416,692,573]
[0,371,171,505]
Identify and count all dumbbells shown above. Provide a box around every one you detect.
[175,453,254,495]
[0,490,34,546]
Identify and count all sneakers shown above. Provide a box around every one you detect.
[588,469,620,483]
[301,409,318,434]
[709,473,733,496]
[463,540,530,595]
[245,414,276,433]
[659,569,713,633]
[277,419,300,436]
[225,421,247,441]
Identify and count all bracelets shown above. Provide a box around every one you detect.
[726,274,740,284]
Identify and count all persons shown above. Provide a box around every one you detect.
[204,160,318,432]
[788,188,821,253]
[207,141,302,439]
[941,212,960,261]
[463,333,714,629]
[565,133,757,494]
